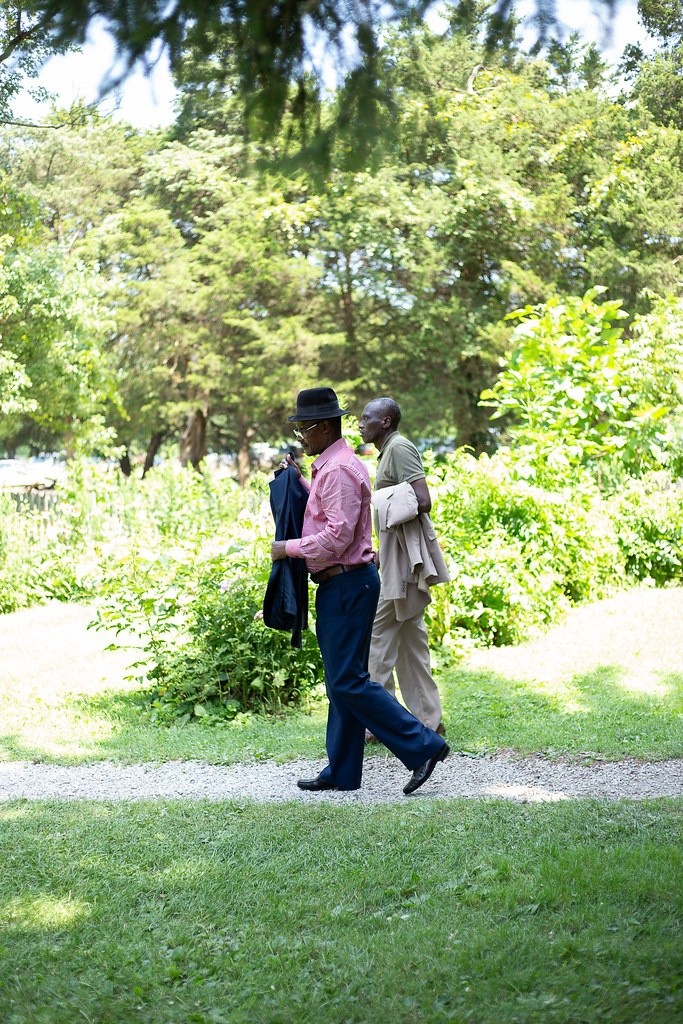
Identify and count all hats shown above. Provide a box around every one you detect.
[287,387,350,422]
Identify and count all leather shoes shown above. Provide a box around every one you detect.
[402,743,451,794]
[296,774,361,790]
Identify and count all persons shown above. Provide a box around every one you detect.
[349,398,448,742]
[266,387,451,793]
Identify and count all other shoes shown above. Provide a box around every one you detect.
[365,728,377,740]
[435,724,446,736]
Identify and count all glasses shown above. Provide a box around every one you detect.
[292,424,316,439]
